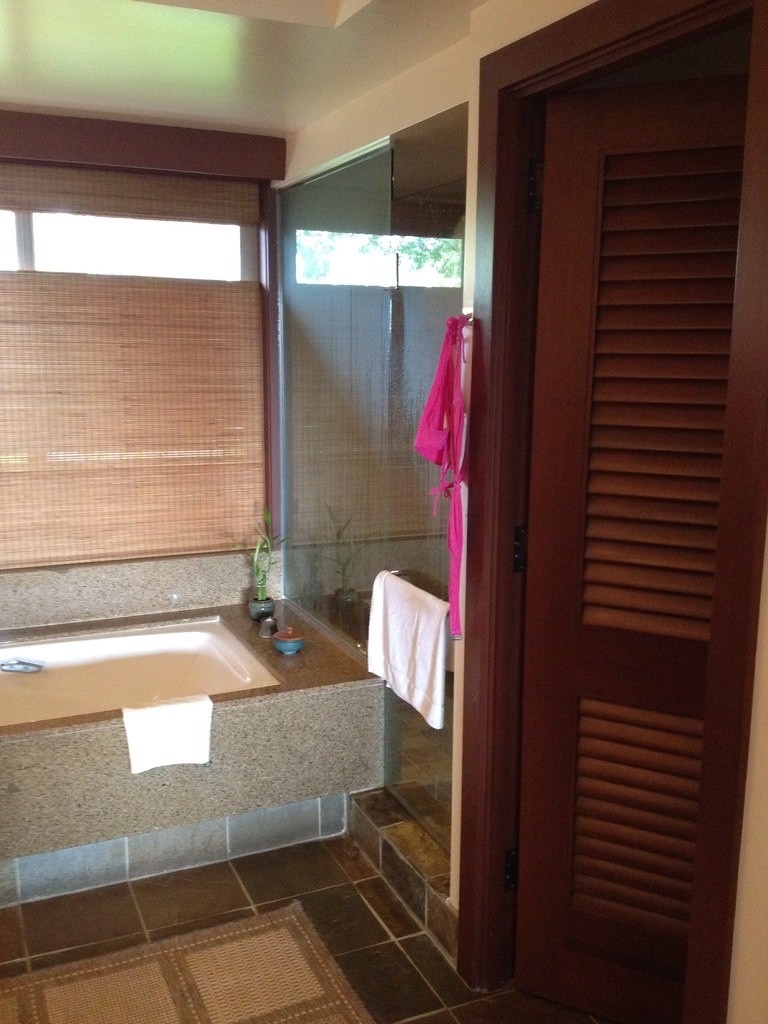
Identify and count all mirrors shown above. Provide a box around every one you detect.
[268,101,471,855]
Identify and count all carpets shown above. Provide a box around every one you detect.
[0,899,378,1024]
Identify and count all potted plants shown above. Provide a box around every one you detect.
[321,503,366,607]
[233,503,289,623]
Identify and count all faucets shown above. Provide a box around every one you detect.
[0,657,44,674]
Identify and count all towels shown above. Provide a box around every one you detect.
[367,570,452,728]
[120,693,214,775]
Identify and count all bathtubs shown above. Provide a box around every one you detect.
[1,596,386,911]
